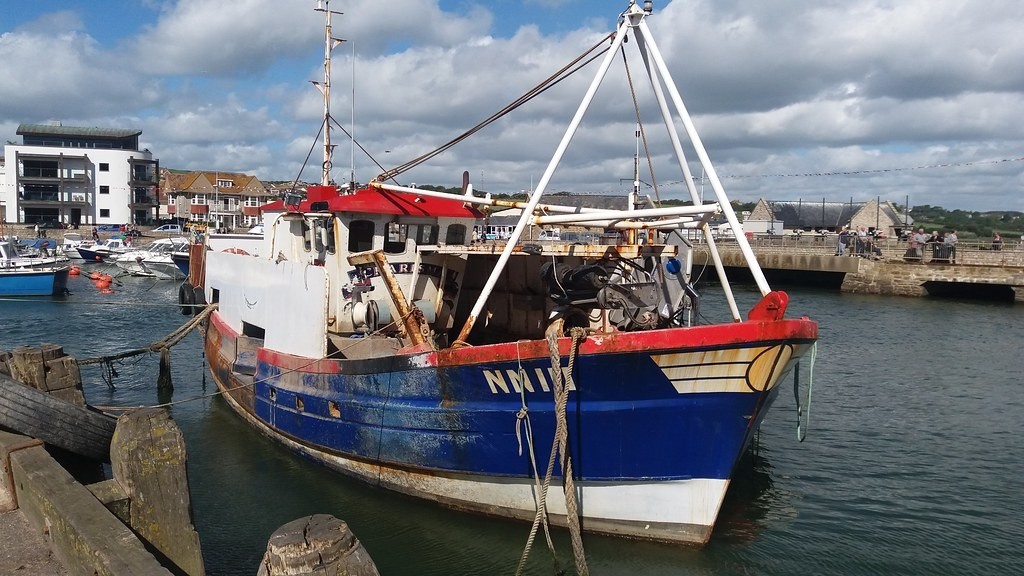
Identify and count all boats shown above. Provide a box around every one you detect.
[0,231,191,297]
[188,1,821,547]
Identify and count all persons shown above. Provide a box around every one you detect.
[834,220,1006,269]
[473,227,486,243]
[92,226,99,239]
[34,222,40,238]
[120,223,143,237]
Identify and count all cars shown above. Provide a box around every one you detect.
[25,218,68,230]
[96,223,129,231]
[150,224,184,232]
